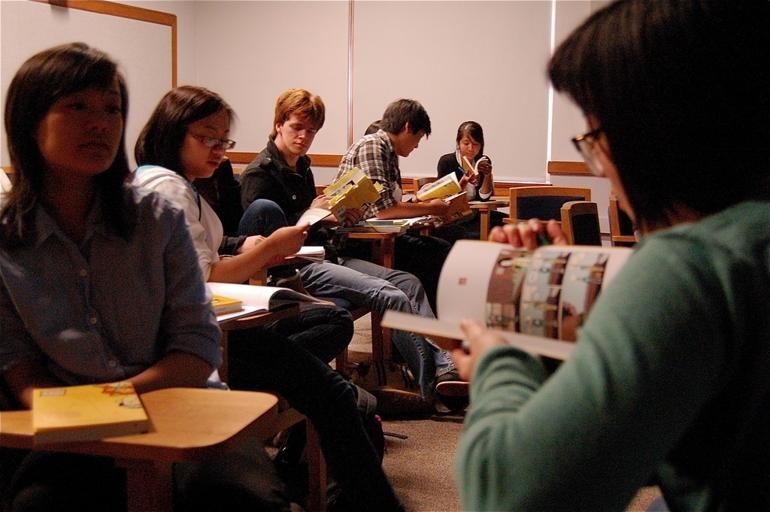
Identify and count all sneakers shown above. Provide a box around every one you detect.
[434,368,471,412]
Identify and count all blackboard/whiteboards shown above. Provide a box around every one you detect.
[1,3,177,170]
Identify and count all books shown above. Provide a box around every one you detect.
[29,377,153,447]
[375,233,639,366]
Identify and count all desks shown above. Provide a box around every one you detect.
[414,200,506,242]
[0,304,332,511]
[247,198,397,366]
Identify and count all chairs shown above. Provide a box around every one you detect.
[509,184,635,249]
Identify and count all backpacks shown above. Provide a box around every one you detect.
[270,379,386,512]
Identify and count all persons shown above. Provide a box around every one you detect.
[1,38,305,511]
[445,0,770,512]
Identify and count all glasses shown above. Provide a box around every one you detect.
[571,125,608,178]
[187,133,237,150]
